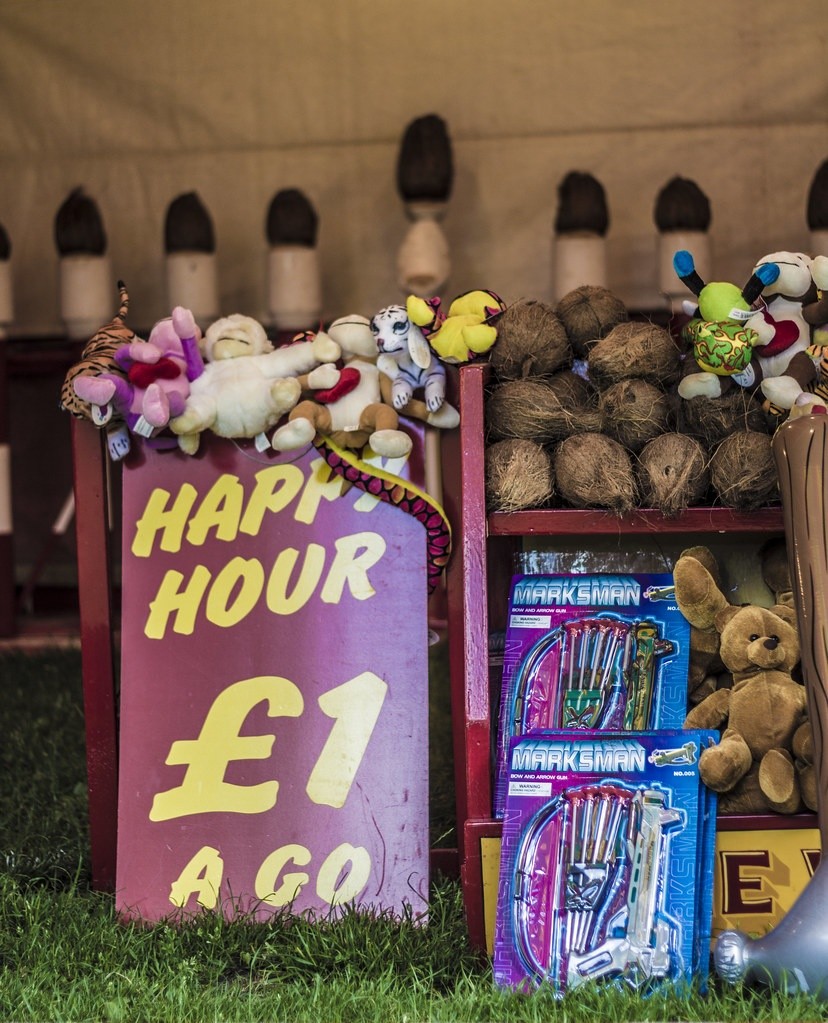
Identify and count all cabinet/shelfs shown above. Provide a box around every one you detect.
[454,364,820,967]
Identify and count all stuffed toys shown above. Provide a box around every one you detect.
[58,278,508,460]
[672,251,827,446]
[671,545,819,816]
